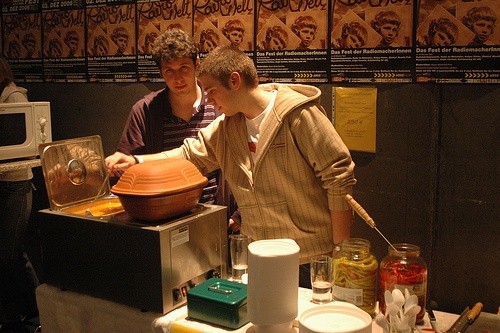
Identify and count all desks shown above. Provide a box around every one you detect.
[35,273,459,333]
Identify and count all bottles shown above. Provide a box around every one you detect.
[332,238,379,317]
[378,243,428,324]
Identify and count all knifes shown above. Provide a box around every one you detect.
[427,304,442,333]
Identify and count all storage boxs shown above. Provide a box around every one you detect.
[187,278,251,328]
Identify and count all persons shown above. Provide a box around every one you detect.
[111,28,242,236]
[104,45,357,294]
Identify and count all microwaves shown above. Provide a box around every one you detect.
[0,101,52,161]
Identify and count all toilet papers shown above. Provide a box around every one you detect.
[248,239,300,325]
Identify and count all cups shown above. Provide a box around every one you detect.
[230,235,249,281]
[310,256,332,304]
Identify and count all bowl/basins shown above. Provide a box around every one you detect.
[110,157,209,222]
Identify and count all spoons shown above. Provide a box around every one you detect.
[374,288,421,333]
